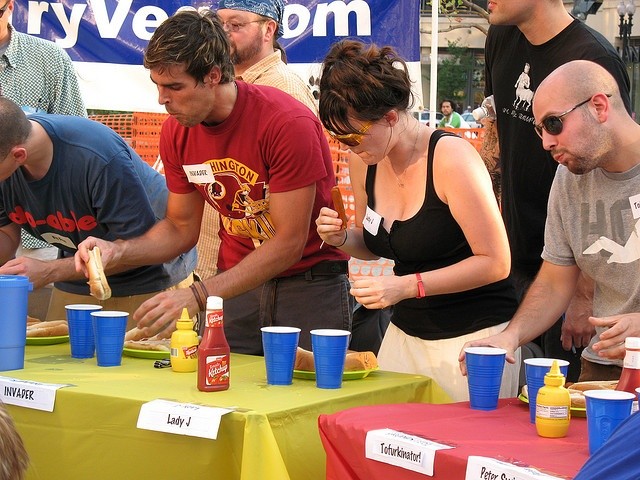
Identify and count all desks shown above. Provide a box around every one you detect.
[0,329,448,480]
[317,396,638,480]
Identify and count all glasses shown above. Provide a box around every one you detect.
[0,0,10,17]
[325,107,397,147]
[534,94,612,140]
[219,19,270,31]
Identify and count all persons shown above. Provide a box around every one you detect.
[459,60,640,382]
[0,95,197,343]
[74,10,351,356]
[195,0,320,282]
[0,0,90,322]
[437,99,478,139]
[315,38,522,403]
[485,1,632,383]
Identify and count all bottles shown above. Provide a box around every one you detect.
[535,361,571,438]
[473,95,497,120]
[615,337,640,400]
[171,308,199,372]
[197,296,231,392]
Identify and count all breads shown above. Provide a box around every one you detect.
[25,317,68,336]
[83,246,112,300]
[124,327,170,349]
[568,380,619,389]
[522,385,586,407]
[294,347,377,371]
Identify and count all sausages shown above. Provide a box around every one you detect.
[331,186,347,230]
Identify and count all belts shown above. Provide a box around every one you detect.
[298,261,348,277]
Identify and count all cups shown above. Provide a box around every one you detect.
[0,274,34,370]
[90,311,129,366]
[260,327,301,386]
[464,347,507,411]
[635,387,640,411]
[582,390,636,457]
[523,357,569,423]
[65,304,102,358]
[310,329,351,388]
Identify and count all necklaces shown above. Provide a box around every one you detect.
[388,119,419,187]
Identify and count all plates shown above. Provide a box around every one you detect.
[292,365,380,381]
[25,335,69,346]
[122,348,171,361]
[518,392,586,418]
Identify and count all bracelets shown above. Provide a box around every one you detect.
[199,281,209,300]
[334,230,347,247]
[189,283,204,312]
[416,273,425,299]
